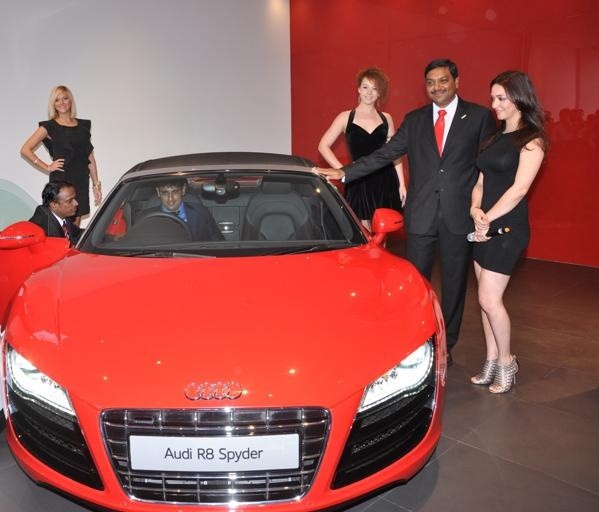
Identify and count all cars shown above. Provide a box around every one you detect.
[1,151,447,511]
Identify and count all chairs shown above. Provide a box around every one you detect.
[143,184,204,242]
[243,178,313,242]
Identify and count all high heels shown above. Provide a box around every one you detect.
[470,358,498,385]
[488,353,520,393]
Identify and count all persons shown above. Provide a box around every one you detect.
[319,68,408,232]
[469,72,551,400]
[317,58,498,368]
[138,180,223,244]
[18,83,103,228]
[24,179,84,250]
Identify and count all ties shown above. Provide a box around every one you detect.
[434,110,447,157]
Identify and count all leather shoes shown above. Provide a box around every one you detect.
[447,351,454,367]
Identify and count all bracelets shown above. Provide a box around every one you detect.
[470,205,478,218]
[32,158,40,165]
[92,182,101,190]
